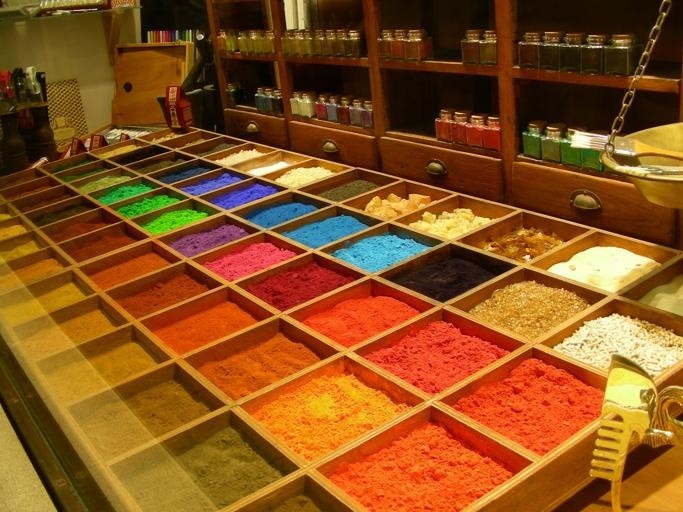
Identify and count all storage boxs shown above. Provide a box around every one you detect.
[54,41,195,158]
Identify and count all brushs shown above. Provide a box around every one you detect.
[569,130,683,161]
[616,165,683,178]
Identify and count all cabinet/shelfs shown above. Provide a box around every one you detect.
[203,1,682,257]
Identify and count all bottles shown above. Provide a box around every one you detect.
[516,30,644,77]
[522,119,609,173]
[376,25,432,60]
[436,108,502,151]
[226,81,247,105]
[462,30,497,65]
[217,27,366,59]
[253,88,374,129]
